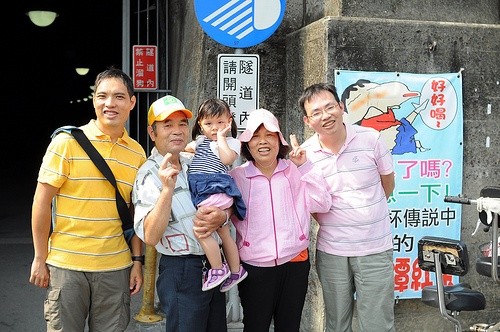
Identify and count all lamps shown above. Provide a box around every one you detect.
[28,11,57,27]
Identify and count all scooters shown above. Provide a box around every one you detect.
[418,186,500,332]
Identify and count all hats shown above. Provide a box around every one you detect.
[146,95,193,126]
[237,108,288,146]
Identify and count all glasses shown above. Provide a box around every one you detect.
[304,101,344,121]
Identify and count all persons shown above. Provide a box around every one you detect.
[131,95,235,332]
[299,82,395,332]
[229,108,333,332]
[29,68,147,332]
[183,97,248,292]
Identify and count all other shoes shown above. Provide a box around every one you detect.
[219,263,248,293]
[201,261,231,292]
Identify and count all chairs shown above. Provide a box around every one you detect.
[475,185,500,284]
[418,235,486,332]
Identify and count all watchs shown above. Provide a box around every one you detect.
[221,211,229,226]
[132,255,145,265]
